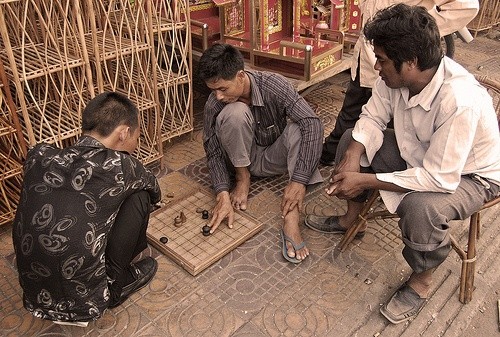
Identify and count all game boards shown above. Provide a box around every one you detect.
[146,185,262,277]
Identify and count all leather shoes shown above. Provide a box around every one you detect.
[379,281,427,324]
[305,214,367,238]
[107,256,158,307]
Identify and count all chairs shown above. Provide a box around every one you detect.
[175,0,365,81]
[338,73,500,304]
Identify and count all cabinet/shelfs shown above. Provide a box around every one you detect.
[0,0,195,225]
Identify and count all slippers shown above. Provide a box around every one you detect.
[281,227,303,263]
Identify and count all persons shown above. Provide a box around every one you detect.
[198,44,324,265]
[12,92,161,321]
[303,4,500,324]
[320,0,481,169]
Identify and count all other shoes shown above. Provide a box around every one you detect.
[319,152,337,165]
